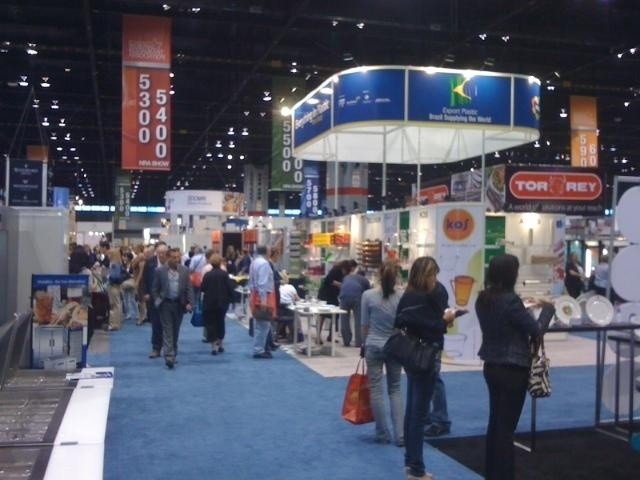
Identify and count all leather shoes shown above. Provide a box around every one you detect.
[166,360,174,370]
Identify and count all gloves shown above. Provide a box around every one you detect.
[360,336,366,357]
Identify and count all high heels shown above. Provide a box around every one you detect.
[136,319,145,325]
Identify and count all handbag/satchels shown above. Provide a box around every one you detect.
[255,305,273,320]
[191,312,204,326]
[109,270,130,284]
[527,321,552,397]
[385,332,436,374]
[342,356,374,424]
[250,319,272,336]
[190,272,201,287]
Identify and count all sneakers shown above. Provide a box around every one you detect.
[407,469,434,480]
[203,339,210,342]
[254,352,273,358]
[219,348,223,352]
[425,423,450,437]
[212,350,217,355]
[149,350,160,358]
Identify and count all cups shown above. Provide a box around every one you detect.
[450,276,476,306]
[441,334,467,357]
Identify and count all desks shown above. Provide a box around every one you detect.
[607,333,639,436]
[288,303,347,357]
[514,321,640,453]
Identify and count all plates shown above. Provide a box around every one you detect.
[553,296,582,325]
[585,295,613,327]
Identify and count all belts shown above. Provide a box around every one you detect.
[164,298,178,303]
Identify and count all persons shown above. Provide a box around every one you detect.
[132,244,147,325]
[423,280,452,437]
[268,247,287,348]
[189,248,206,308]
[225,245,236,273]
[360,263,405,447]
[185,251,194,266]
[191,243,198,250]
[155,241,167,249]
[200,254,235,355]
[69,240,123,331]
[143,245,168,358]
[393,256,454,480]
[564,251,584,299]
[150,249,193,367]
[315,259,358,344]
[201,249,228,343]
[594,255,615,306]
[337,266,370,347]
[121,252,140,319]
[279,269,302,341]
[249,244,276,358]
[120,245,131,255]
[237,248,251,276]
[474,254,555,480]
